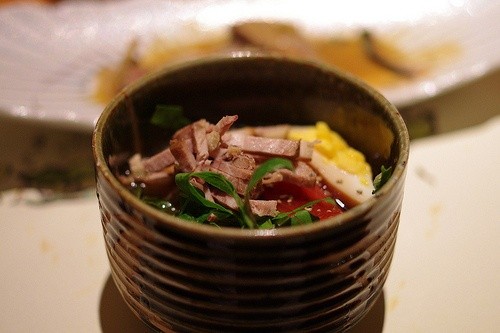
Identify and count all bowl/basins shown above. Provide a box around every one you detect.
[93,52,411,333]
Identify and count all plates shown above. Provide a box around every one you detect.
[0,0,500,135]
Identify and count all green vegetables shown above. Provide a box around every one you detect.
[124,104,338,231]
